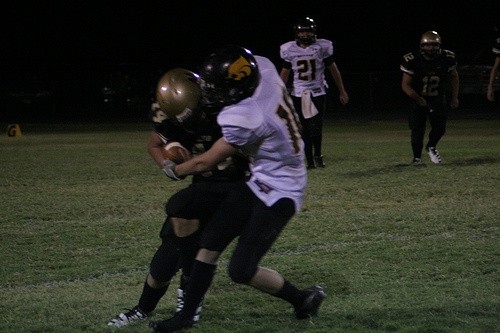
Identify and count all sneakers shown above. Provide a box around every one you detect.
[107,305,154,329]
[176,289,201,322]
[314,155,326,168]
[428,147,443,164]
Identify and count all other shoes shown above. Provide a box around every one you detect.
[149,312,194,333]
[295,285,326,319]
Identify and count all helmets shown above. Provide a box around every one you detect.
[293,16,317,45]
[420,31,441,57]
[157,67,207,125]
[199,45,260,115]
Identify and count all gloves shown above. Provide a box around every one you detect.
[161,159,187,181]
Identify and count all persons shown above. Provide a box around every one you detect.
[105,66,264,328]
[486,30,500,127]
[279,15,349,170]
[143,44,326,333]
[400,30,458,165]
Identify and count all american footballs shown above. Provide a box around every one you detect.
[166,142,188,182]
